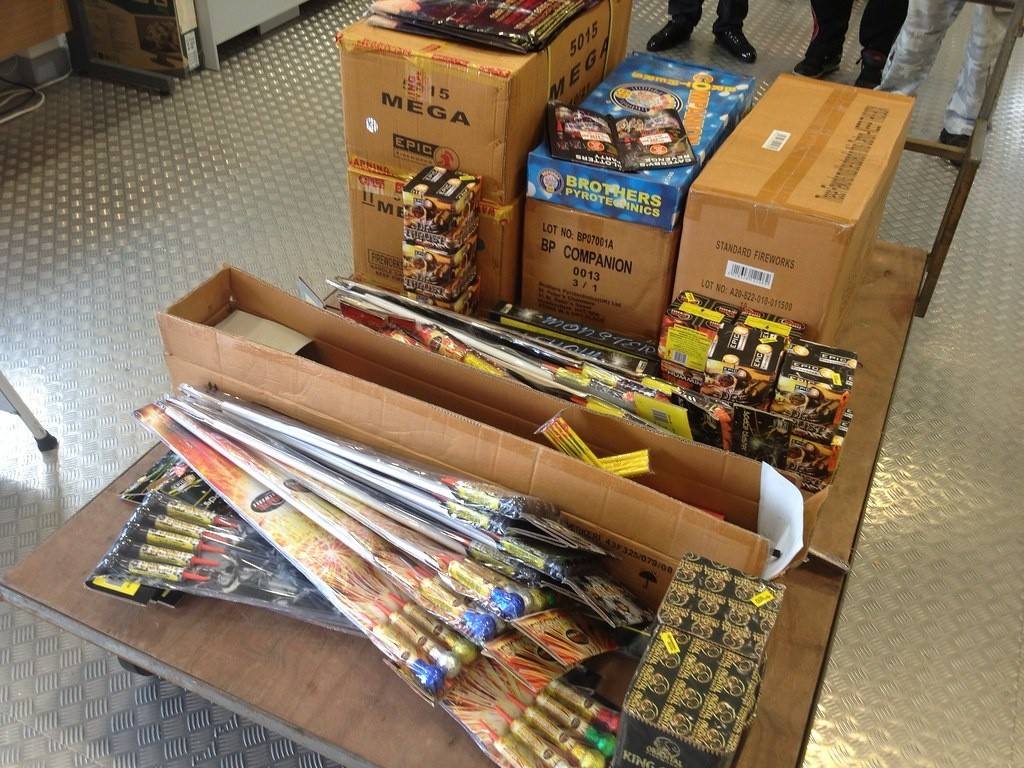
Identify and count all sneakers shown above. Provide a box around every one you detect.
[792,49,841,78]
[853,48,886,89]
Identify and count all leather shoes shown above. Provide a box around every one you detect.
[714,29,757,62]
[646,19,693,51]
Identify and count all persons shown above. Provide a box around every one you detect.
[793,0,910,89]
[873,0,1024,169]
[646,0,757,64]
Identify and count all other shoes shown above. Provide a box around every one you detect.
[940,127,972,167]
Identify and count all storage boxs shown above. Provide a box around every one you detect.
[0,31,73,90]
[334,0,916,490]
[73,0,204,78]
[154,263,833,600]
